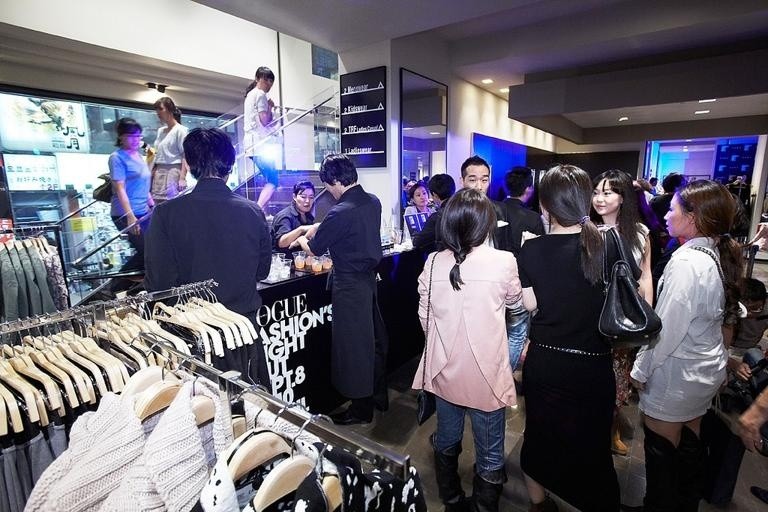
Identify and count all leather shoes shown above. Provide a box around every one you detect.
[328,404,373,425]
[374,398,389,412]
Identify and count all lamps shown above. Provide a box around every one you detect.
[157,83,169,94]
[142,78,157,91]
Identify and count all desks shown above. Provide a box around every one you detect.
[249,211,507,417]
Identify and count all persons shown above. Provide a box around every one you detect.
[153,97,188,209]
[141,126,274,398]
[109,117,154,294]
[244,65,278,221]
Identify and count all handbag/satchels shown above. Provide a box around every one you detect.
[597,226,662,351]
[93,172,113,203]
[416,390,436,426]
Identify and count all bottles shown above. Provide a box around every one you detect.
[381,239,394,256]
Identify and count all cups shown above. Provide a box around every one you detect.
[268,251,332,281]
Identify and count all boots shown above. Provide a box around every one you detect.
[470,464,508,512]
[527,491,560,511]
[429,432,472,512]
[621,422,702,512]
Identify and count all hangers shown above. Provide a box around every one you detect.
[0,278,344,512]
[0,222,58,254]
